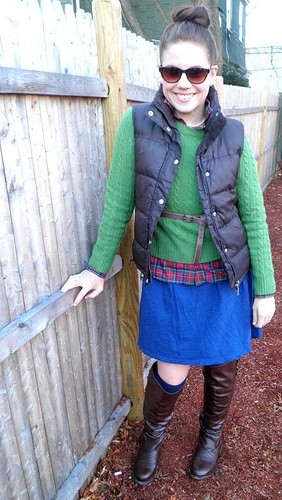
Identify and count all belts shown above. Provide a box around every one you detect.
[161,212,208,263]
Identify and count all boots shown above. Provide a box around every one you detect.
[190,361,236,478]
[132,361,184,485]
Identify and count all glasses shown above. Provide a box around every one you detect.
[159,65,211,84]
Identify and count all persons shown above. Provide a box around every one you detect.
[62,7,276,484]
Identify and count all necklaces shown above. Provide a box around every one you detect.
[185,119,205,126]
